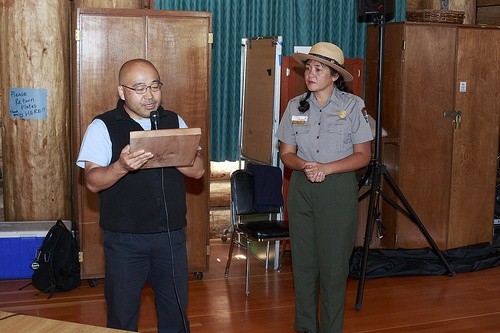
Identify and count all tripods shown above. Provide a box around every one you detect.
[358,22,456,310]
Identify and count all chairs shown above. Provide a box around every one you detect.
[224,166,291,297]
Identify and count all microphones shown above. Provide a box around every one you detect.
[151,111,159,131]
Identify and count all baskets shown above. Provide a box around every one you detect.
[407,9,465,24]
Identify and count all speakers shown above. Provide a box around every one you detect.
[356,0,396,23]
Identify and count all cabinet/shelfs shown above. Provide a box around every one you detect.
[69,8,214,286]
[367,21,500,251]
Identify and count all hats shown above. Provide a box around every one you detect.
[292,42,354,81]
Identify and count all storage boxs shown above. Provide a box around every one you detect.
[0,219,72,280]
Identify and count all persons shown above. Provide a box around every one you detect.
[275,43,373,333]
[77,59,205,333]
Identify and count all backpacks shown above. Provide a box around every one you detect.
[19,220,80,299]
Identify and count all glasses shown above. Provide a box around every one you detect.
[120,82,163,94]
